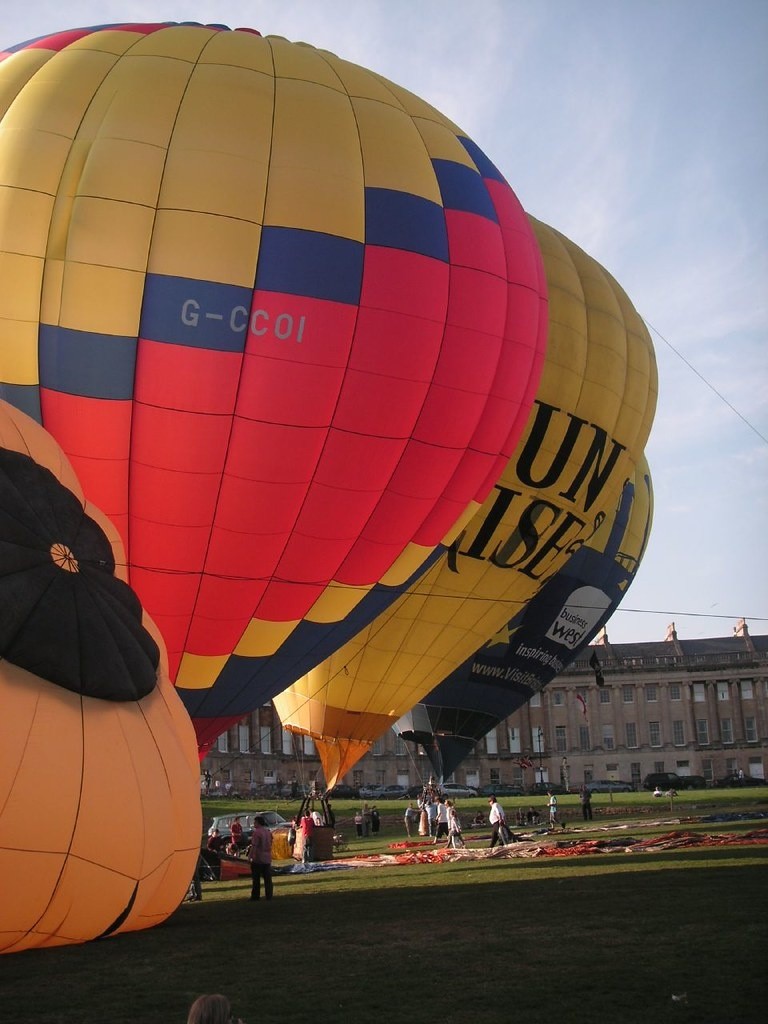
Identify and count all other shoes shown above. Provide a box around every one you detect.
[184,894,195,901]
[561,822,566,829]
[188,895,202,902]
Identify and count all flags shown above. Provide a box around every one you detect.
[575,693,587,715]
[589,650,605,686]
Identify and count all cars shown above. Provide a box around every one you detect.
[405,787,423,800]
[679,775,706,790]
[711,773,767,788]
[531,781,563,794]
[435,782,479,801]
[375,785,409,802]
[323,785,358,799]
[358,783,382,799]
[583,779,633,793]
[612,781,636,792]
[281,783,310,799]
[482,784,524,797]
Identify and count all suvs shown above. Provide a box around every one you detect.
[642,773,684,793]
[208,811,298,843]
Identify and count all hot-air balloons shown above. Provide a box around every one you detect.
[387,447,655,835]
[0,20,551,772]
[271,210,658,866]
[0,389,208,969]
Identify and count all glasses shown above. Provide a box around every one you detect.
[488,801,491,803]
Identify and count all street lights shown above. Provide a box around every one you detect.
[537,725,545,796]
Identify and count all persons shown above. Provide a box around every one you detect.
[488,795,507,848]
[355,803,381,839]
[474,810,488,824]
[417,793,466,849]
[653,787,662,797]
[515,806,541,826]
[546,789,566,830]
[404,803,423,837]
[733,768,744,780]
[207,816,274,899]
[184,861,203,902]
[579,784,592,821]
[292,804,335,864]
